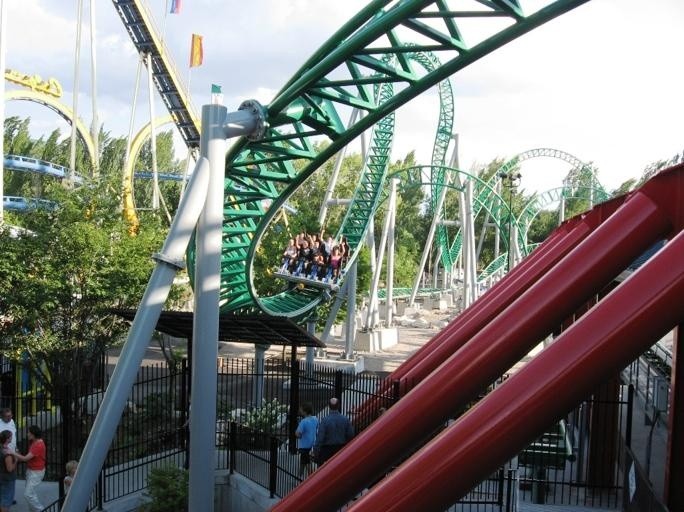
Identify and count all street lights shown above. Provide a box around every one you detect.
[499,173,522,273]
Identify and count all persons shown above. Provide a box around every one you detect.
[316,399,355,462]
[179,390,191,471]
[1,428,20,511]
[292,400,319,486]
[62,462,78,498]
[2,426,50,512]
[0,407,19,462]
[279,229,350,285]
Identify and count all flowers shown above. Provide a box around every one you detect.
[243,396,286,433]
